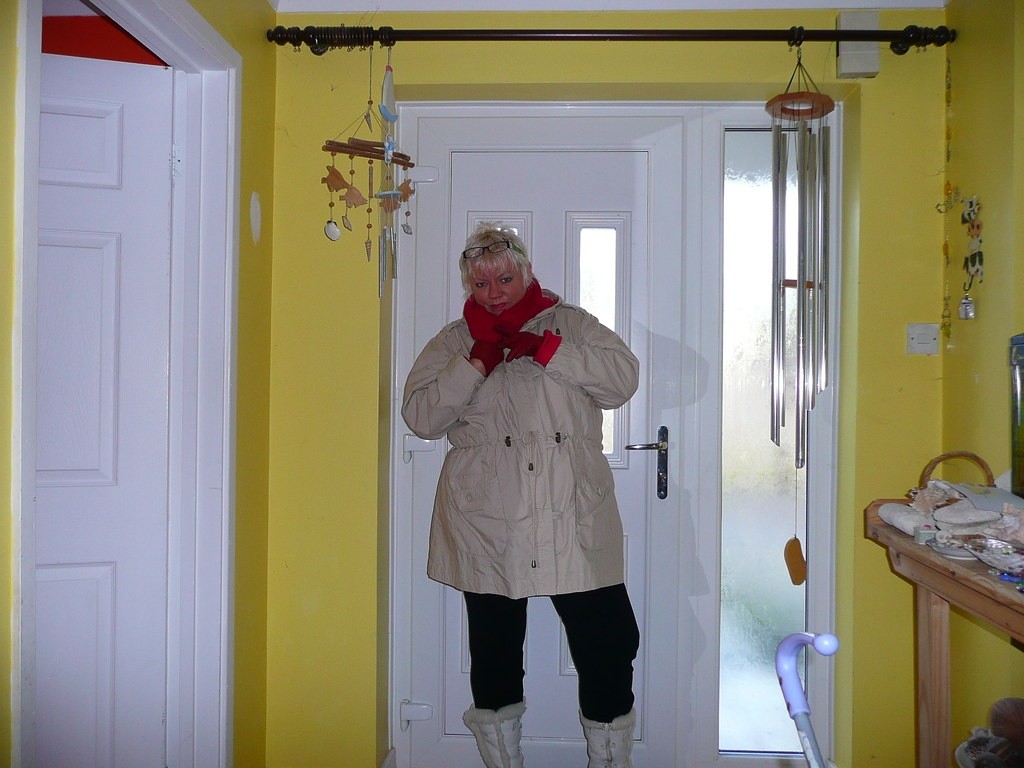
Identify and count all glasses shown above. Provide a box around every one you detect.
[463,240,525,262]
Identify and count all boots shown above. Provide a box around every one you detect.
[578,707,636,768]
[462,696,527,768]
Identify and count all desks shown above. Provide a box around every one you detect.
[865,499,1024,768]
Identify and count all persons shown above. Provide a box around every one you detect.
[400,224,640,768]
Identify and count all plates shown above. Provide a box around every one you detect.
[926,534,998,561]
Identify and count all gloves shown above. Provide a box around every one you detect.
[469,332,504,377]
[494,326,544,363]
